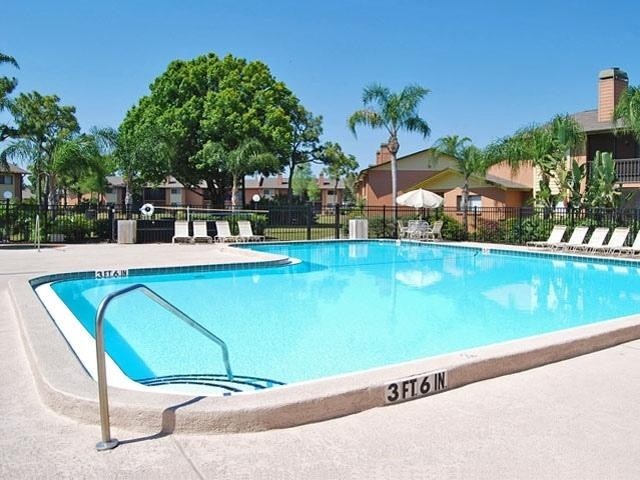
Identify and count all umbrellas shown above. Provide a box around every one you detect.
[395,188,443,209]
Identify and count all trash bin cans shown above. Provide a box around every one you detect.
[348,218,368,239]
[117,220,137,243]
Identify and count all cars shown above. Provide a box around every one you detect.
[327,202,355,215]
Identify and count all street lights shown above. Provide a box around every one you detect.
[252,194,260,235]
[3,191,12,240]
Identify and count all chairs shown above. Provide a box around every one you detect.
[192,220,212,244]
[237,220,265,242]
[215,220,238,243]
[397,219,444,242]
[172,219,193,244]
[526,224,640,259]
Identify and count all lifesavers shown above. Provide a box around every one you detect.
[141,203,154,215]
